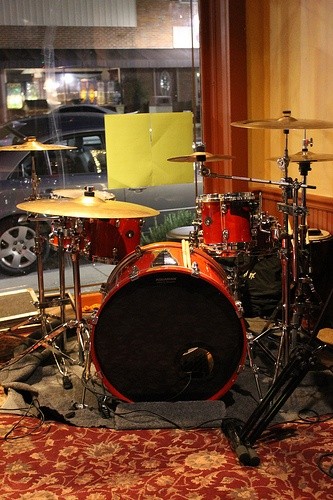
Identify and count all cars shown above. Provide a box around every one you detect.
[11,103,117,125]
[0,113,204,276]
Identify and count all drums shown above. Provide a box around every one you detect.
[195,189,262,257]
[88,217,143,266]
[48,188,117,254]
[88,240,248,403]
[290,227,333,279]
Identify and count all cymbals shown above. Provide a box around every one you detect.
[16,193,160,218]
[264,149,333,162]
[166,151,238,163]
[0,139,78,152]
[230,115,333,130]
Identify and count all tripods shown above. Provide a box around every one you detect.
[198,131,333,404]
[0,151,92,389]
